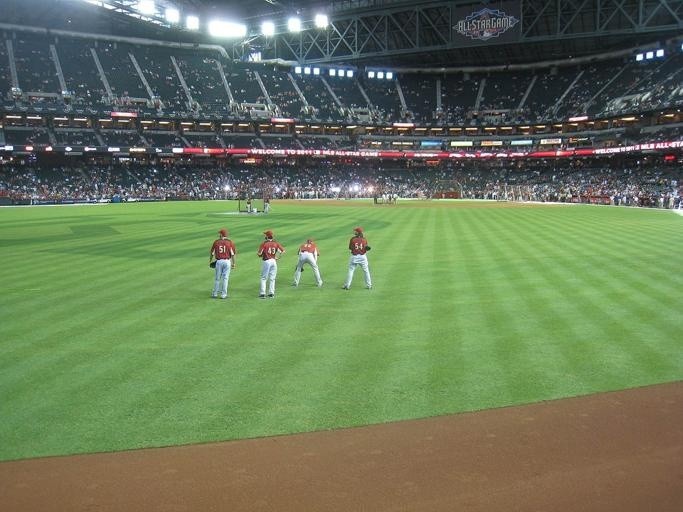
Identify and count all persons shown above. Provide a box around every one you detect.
[341,227,373,290]
[0,38,683,214]
[209,230,236,299]
[257,230,285,299]
[291,239,324,287]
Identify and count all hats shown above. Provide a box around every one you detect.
[353,228,363,233]
[218,228,227,237]
[264,231,272,237]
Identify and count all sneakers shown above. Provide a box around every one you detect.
[268,294,274,298]
[258,294,266,299]
